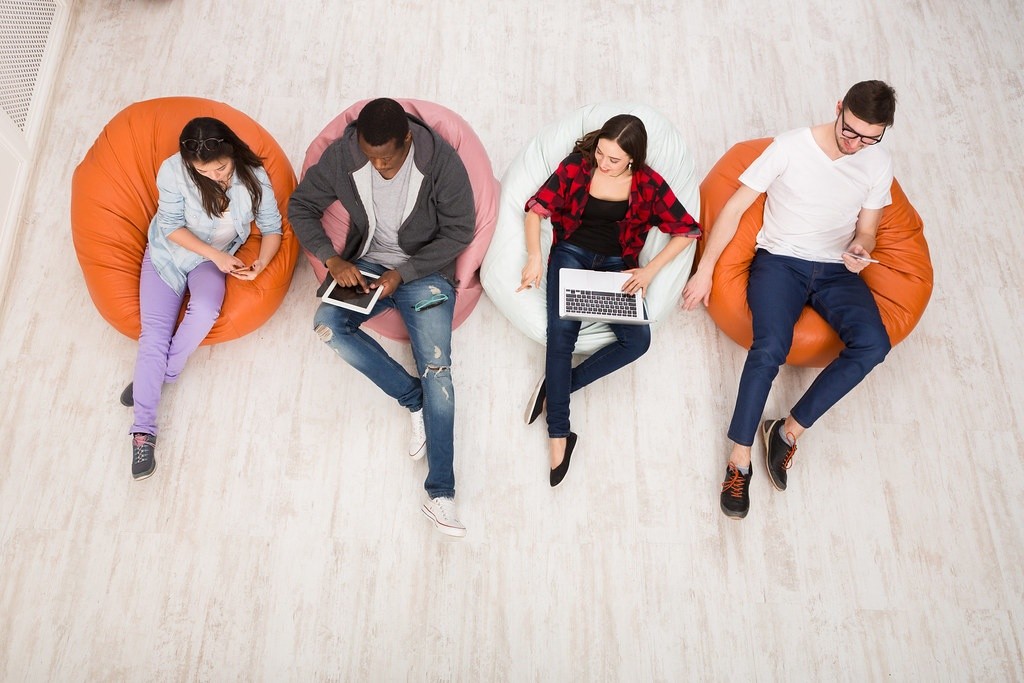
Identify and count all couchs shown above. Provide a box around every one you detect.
[480,96,702,358]
[71,94,302,346]
[689,136,935,370]
[293,98,503,343]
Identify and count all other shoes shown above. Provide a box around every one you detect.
[526,373,578,491]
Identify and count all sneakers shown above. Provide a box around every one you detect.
[408,409,466,537]
[721,418,797,520]
[120,380,157,481]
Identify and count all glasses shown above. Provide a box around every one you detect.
[180,137,224,154]
[841,108,886,146]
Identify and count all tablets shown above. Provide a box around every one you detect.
[321,269,385,315]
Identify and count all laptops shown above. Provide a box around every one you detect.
[559,268,656,326]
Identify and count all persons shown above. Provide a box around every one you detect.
[283,98,477,538]
[119,116,282,481]
[682,78,896,521]
[514,115,704,487]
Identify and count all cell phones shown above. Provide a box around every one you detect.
[231,266,251,273]
[843,252,879,263]
